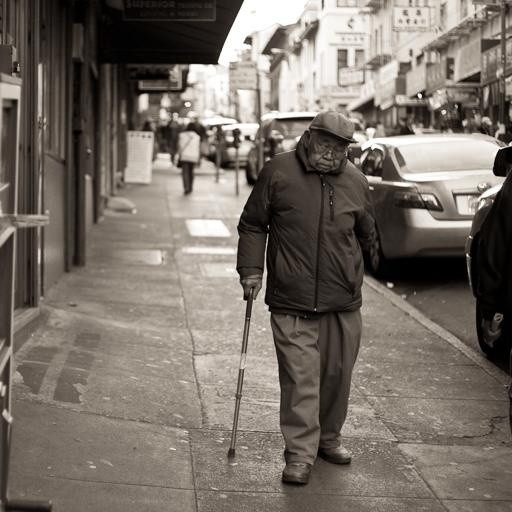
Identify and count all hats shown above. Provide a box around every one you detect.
[309,109,359,143]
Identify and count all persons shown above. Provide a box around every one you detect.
[177,122,201,197]
[237,111,380,486]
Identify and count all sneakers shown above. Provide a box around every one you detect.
[319,443,352,465]
[282,462,311,485]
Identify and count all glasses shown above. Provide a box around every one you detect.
[314,142,348,161]
